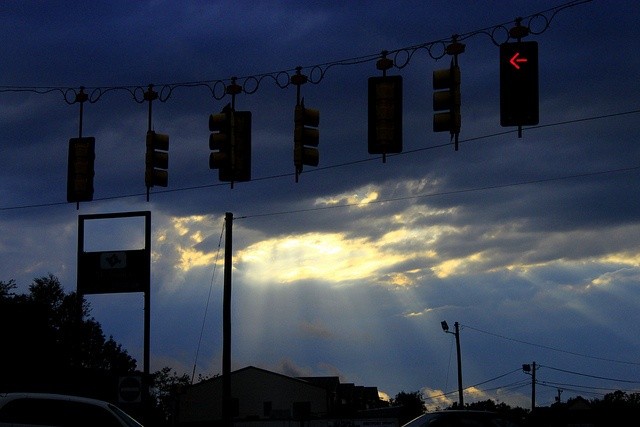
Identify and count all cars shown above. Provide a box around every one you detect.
[2,392,143,425]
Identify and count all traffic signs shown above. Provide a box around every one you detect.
[368,59,403,161]
[219,110,251,181]
[67,137,94,202]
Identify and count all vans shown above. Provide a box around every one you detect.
[402,410,499,427]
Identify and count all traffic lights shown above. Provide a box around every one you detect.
[209,113,226,169]
[294,109,319,167]
[146,132,169,186]
[500,41,539,137]
[433,69,460,132]
